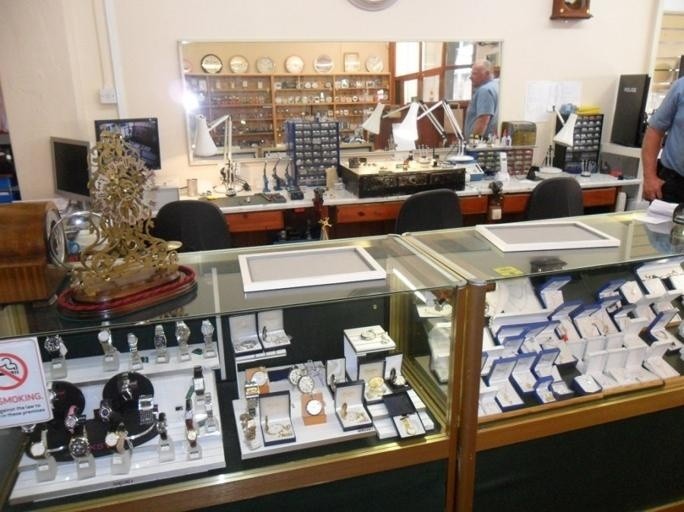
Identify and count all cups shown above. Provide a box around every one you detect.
[581,159,596,177]
[187,179,198,197]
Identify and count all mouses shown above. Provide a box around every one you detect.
[66,213,87,228]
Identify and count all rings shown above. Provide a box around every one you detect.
[362,329,376,341]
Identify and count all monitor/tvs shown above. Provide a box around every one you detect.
[50,135,91,213]
[94,117,161,170]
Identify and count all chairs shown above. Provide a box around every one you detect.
[525,176,584,221]
[397,189,462,234]
[153,201,232,253]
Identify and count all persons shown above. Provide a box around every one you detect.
[462,60,501,140]
[642,74,684,206]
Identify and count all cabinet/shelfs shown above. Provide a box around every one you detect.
[184,71,392,163]
[0,208,684,512]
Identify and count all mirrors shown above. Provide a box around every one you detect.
[179,38,503,166]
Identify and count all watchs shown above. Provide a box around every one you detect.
[21,320,215,467]
[233,364,324,446]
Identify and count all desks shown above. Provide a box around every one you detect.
[179,173,628,233]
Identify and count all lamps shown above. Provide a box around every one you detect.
[394,96,467,156]
[361,98,450,148]
[192,114,251,197]
[538,104,578,173]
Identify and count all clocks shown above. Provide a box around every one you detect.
[182,52,384,74]
